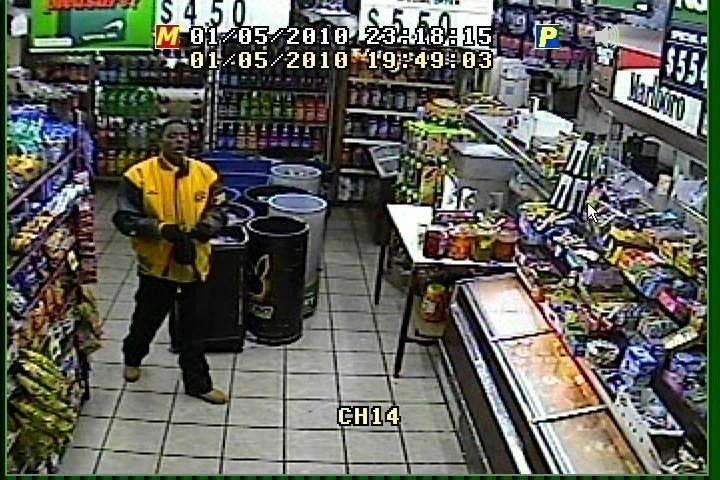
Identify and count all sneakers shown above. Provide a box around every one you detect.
[124,366,139,381]
[199,388,225,404]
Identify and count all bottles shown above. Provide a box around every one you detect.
[59,54,460,175]
[417,283,450,338]
[422,219,518,262]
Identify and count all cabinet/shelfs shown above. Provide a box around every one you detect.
[5,38,472,476]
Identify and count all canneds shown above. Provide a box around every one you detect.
[421,227,495,322]
[339,63,452,201]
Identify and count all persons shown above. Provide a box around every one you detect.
[112,120,227,405]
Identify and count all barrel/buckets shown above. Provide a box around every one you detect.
[216,161,327,346]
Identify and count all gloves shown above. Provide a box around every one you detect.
[191,224,213,244]
[161,223,185,240]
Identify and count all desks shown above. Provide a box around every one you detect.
[374,204,518,376]
